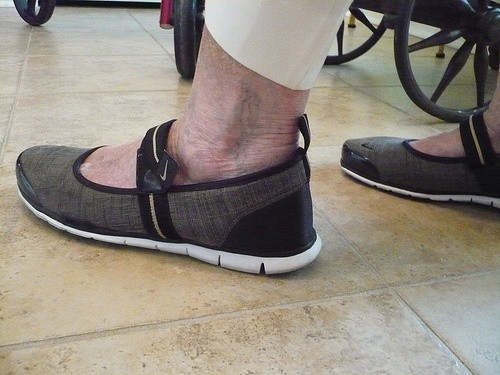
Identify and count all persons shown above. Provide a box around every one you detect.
[17,1,499,276]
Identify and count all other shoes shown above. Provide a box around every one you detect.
[14,112,324,276]
[339,103,499,210]
